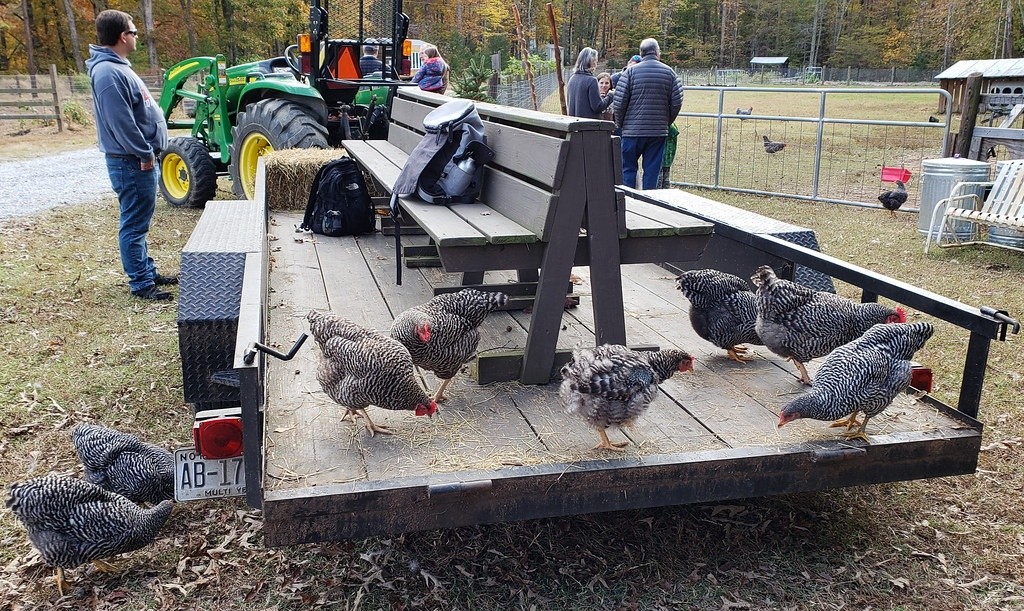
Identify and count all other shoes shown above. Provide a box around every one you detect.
[131,284,174,302]
[153,273,180,286]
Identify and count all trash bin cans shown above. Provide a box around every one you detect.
[918,154,991,240]
[989,160,1024,247]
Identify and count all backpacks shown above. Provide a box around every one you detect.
[300,157,376,237]
[388,98,495,223]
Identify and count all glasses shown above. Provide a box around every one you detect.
[119,29,138,36]
[653,50,662,55]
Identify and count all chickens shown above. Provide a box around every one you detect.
[5,475,175,599]
[763,136,787,154]
[674,270,766,367]
[749,265,908,387]
[389,288,510,404]
[558,340,696,454]
[67,423,177,503]
[305,309,438,438]
[735,107,753,120]
[928,116,940,127]
[877,180,909,219]
[777,323,934,444]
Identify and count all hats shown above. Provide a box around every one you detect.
[362,38,380,51]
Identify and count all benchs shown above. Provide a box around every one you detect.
[925,157,1024,256]
[342,90,716,386]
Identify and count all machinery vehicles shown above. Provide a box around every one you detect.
[156,0,421,209]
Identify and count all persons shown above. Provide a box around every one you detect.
[359,38,391,77]
[614,38,683,189]
[410,44,450,95]
[611,55,642,137]
[595,72,614,121]
[84,10,179,303]
[566,47,616,119]
[656,123,680,189]
